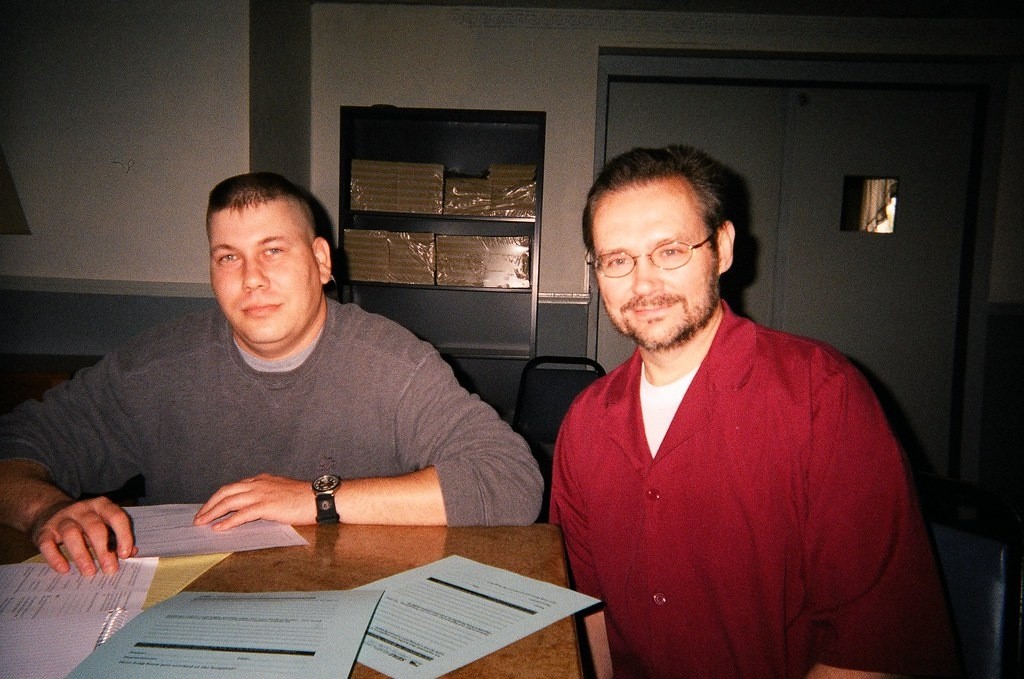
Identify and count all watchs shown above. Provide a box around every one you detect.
[312,473,342,526]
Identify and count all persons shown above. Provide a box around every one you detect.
[0,171,544,577]
[547,144,957,679]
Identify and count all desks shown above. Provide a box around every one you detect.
[0,522,585,679]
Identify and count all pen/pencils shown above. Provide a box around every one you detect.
[107,526,117,553]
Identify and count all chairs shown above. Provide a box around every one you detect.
[513,356,607,524]
[927,521,1008,679]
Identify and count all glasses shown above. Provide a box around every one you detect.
[585,230,715,278]
